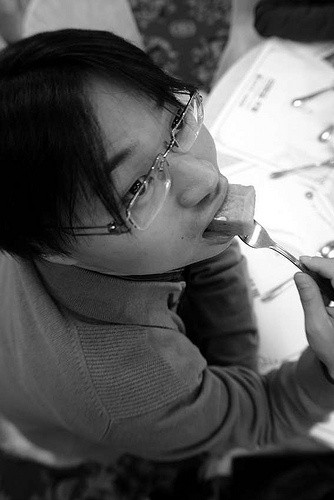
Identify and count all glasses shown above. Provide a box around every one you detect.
[45,86,204,237]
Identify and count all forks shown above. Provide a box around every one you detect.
[238,219,334,302]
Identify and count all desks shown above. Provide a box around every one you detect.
[195,35,333,457]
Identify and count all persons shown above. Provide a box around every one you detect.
[0,28,334,500]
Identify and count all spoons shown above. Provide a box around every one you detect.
[259,239,333,302]
